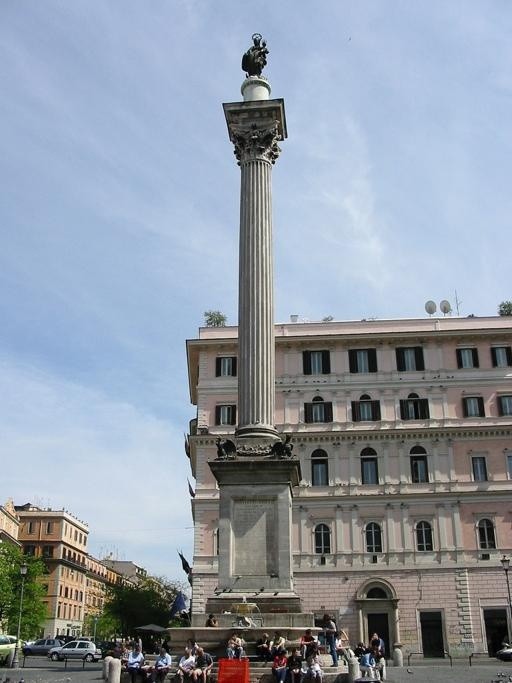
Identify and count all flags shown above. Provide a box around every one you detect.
[180,555,189,573]
[169,592,186,617]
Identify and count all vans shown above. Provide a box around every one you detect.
[75,635,100,642]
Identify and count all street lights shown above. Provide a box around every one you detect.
[500,553,512,619]
[11,557,31,670]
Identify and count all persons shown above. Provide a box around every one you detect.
[113,608,386,683]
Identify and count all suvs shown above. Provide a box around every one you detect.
[55,634,75,643]
[21,638,64,656]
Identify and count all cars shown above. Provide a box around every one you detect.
[1,633,26,667]
[47,639,103,663]
[496,612,511,662]
